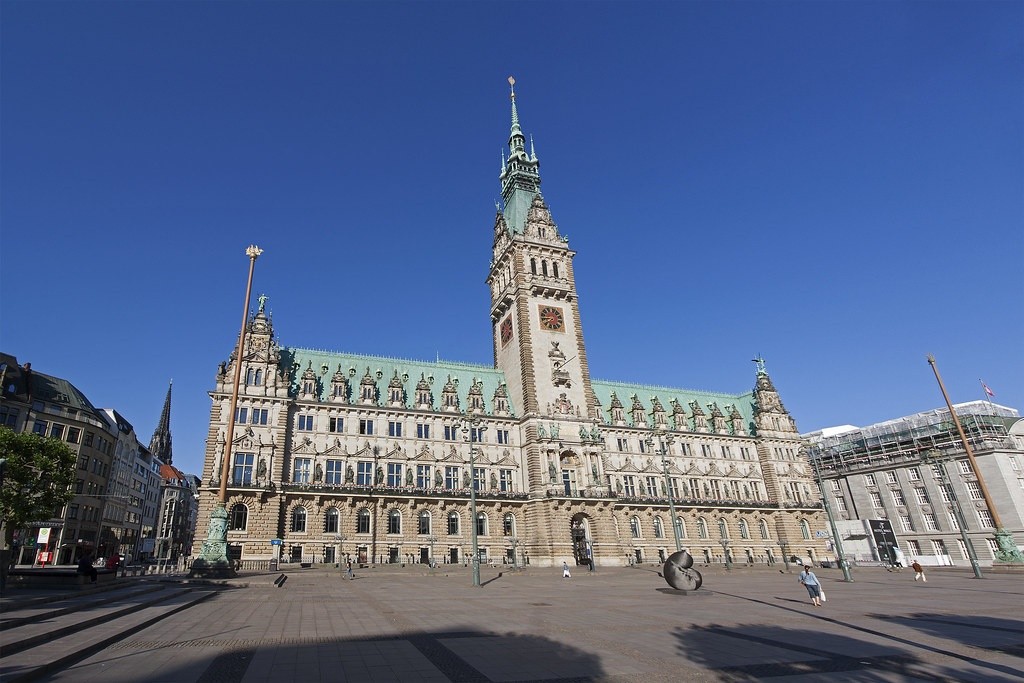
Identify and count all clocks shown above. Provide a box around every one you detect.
[500,312,514,348]
[539,305,566,333]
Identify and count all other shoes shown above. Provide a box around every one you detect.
[814,603,822,607]
[914,577,917,581]
[350,577,354,579]
[342,576,345,580]
[924,581,927,583]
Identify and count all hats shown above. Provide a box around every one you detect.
[349,558,351,560]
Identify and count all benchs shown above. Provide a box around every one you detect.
[6,568,118,585]
[121,565,178,577]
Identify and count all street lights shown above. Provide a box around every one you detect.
[796,444,854,582]
[919,448,983,579]
[644,429,683,552]
[452,410,488,588]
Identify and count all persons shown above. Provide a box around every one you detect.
[563,561,571,577]
[912,560,928,583]
[342,558,355,580]
[797,565,822,606]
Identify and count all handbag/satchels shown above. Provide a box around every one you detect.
[800,580,804,585]
[819,587,826,602]
